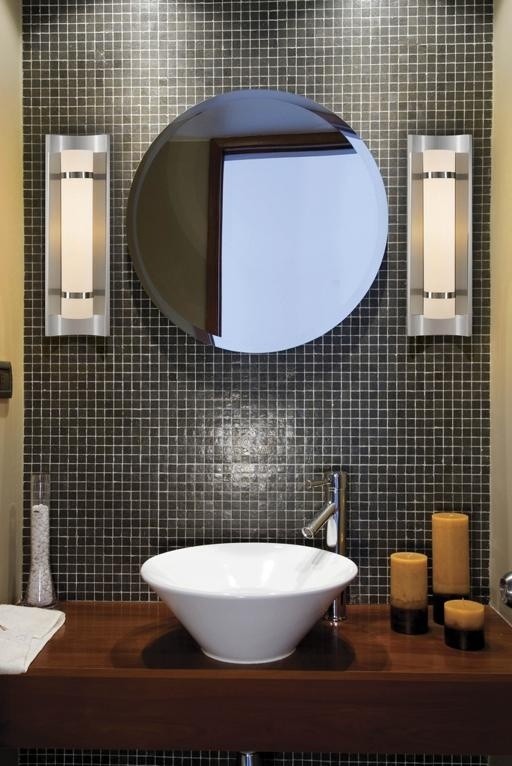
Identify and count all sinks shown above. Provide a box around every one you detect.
[139,543,359,664]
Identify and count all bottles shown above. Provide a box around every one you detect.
[24,474,56,607]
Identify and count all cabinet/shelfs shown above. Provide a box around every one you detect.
[0,598,512,766]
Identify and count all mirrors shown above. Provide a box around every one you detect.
[126,85,390,358]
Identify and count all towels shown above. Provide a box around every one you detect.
[0,601,66,675]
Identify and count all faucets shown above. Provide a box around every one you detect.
[303,470,350,622]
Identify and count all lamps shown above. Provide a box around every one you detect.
[405,133,473,337]
[44,132,111,338]
